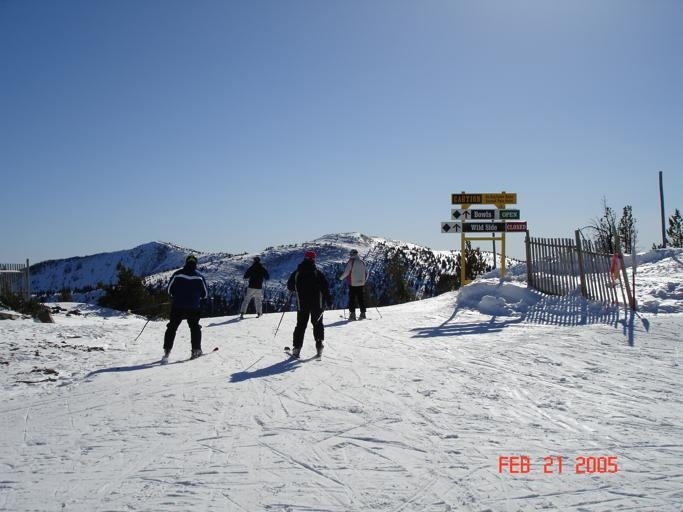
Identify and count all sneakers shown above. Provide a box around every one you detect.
[292,348,323,358]
[161,351,202,363]
[349,312,366,319]
[240,313,262,320]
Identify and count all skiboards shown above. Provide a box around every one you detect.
[339,316,371,321]
[239,314,262,320]
[284,347,322,361]
[160,348,218,365]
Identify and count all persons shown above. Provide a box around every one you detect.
[287,251,331,357]
[161,255,208,365]
[337,249,369,321]
[240,257,270,317]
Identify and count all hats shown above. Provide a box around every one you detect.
[186,255,198,266]
[304,250,316,259]
